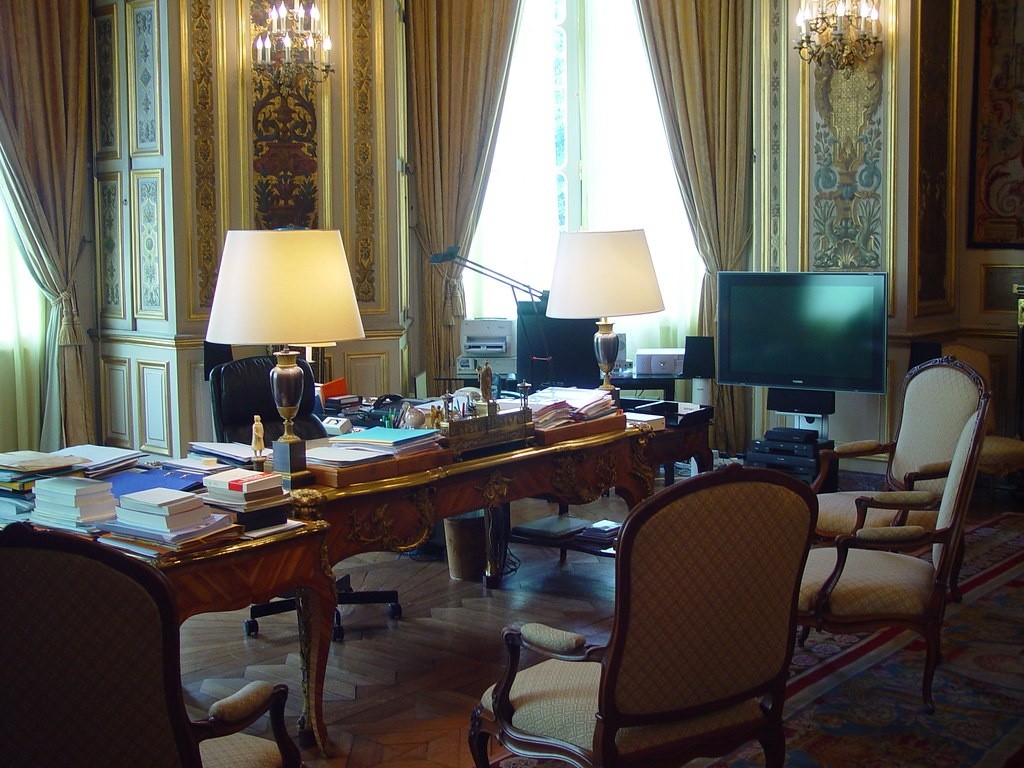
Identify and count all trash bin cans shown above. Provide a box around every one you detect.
[443,515,484,581]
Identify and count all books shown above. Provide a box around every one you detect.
[202,468,290,513]
[328,426,441,448]
[576,520,620,544]
[115,486,233,542]
[0,447,150,516]
[30,475,119,533]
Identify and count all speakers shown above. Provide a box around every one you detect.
[908,341,942,373]
[682,336,716,380]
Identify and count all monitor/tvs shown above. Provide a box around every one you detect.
[716,271,887,395]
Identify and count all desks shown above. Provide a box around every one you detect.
[440,375,692,488]
[1,480,344,756]
[257,421,655,746]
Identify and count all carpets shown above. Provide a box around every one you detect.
[475,473,1023,767]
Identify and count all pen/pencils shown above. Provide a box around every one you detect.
[454,398,465,419]
[379,406,404,428]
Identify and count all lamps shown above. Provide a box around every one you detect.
[793,0,883,70]
[257,0,335,82]
[545,229,664,387]
[205,230,367,488]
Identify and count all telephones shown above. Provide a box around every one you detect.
[367,393,433,420]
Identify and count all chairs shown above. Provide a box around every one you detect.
[211,353,403,641]
[467,467,819,768]
[976,437,1024,515]
[808,355,987,601]
[798,391,993,713]
[0,520,304,767]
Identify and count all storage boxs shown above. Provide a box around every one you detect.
[635,347,686,374]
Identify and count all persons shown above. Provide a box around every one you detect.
[433,405,442,426]
[494,373,502,399]
[251,415,264,459]
[476,359,492,401]
